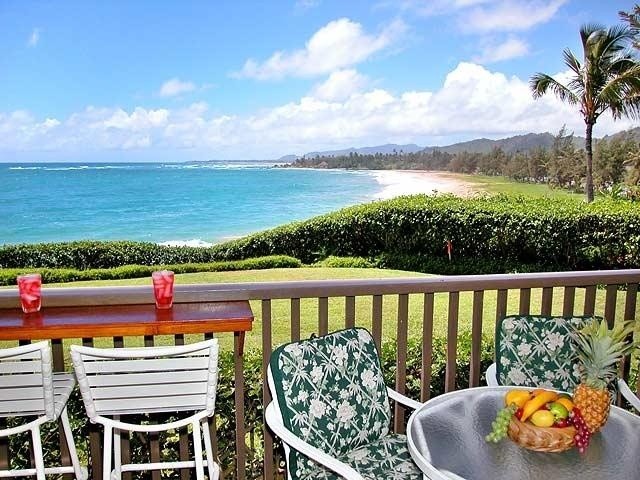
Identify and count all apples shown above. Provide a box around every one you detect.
[548,401,568,420]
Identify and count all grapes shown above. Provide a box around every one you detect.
[486,402,519,444]
[566,407,590,455]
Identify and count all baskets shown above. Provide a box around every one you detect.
[506,414,576,453]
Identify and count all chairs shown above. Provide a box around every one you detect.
[0,341,86,480]
[262,324,432,478]
[486,312,638,414]
[70,338,224,480]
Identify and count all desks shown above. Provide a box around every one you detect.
[408,385,640,479]
[0,299,255,480]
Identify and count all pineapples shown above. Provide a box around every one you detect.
[563,316,640,435]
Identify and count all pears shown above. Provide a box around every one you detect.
[505,389,533,408]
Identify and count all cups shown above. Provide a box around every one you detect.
[16,274,43,313]
[155,309,173,322]
[152,270,175,310]
[22,313,44,329]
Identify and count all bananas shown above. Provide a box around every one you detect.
[520,391,558,422]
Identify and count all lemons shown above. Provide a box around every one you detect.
[530,411,556,426]
[557,397,575,411]
